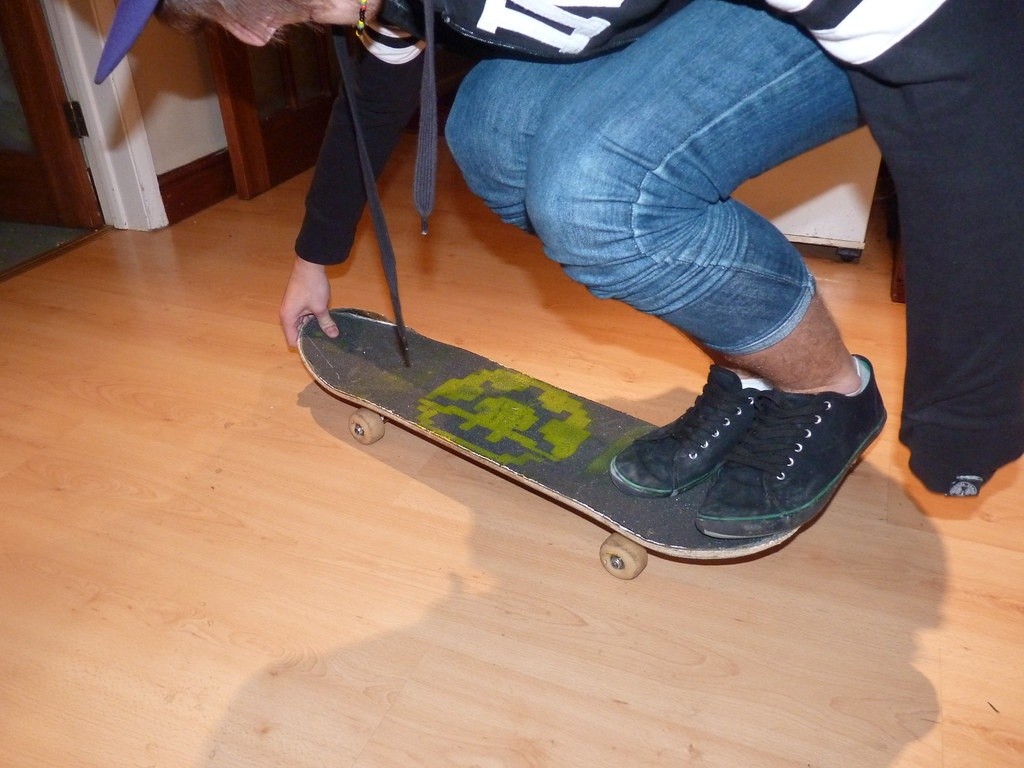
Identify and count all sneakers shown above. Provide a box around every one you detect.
[696,354,888,540]
[609,363,777,498]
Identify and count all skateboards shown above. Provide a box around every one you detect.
[297,306,801,583]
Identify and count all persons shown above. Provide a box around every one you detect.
[92,0,1023,541]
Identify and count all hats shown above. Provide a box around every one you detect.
[92,1,160,84]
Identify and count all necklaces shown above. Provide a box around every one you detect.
[355,0,368,40]
